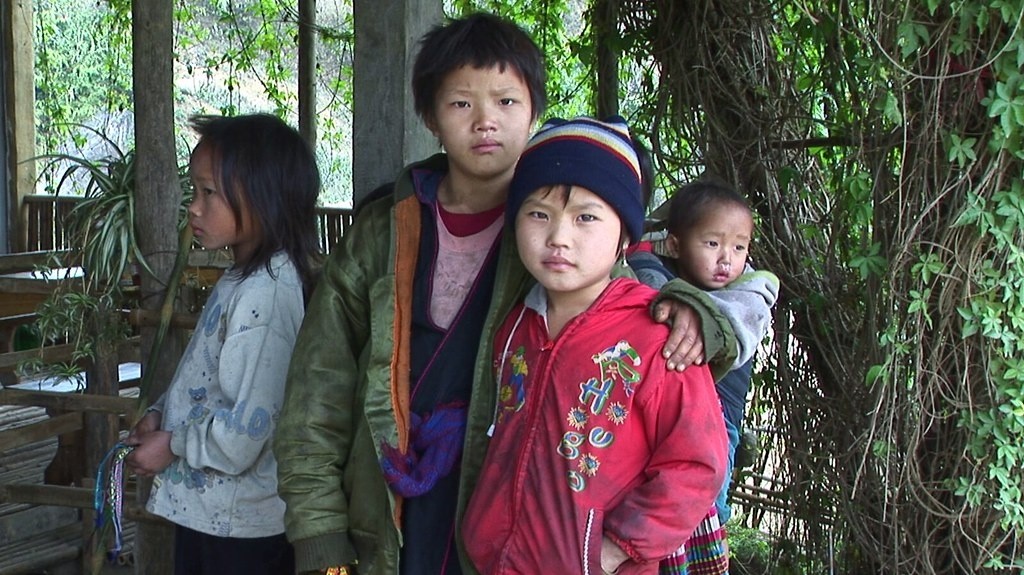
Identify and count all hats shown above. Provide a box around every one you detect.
[507,115,644,247]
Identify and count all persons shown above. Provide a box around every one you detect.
[461,115,729,574]
[272,10,737,575]
[650,176,781,541]
[124,113,326,575]
